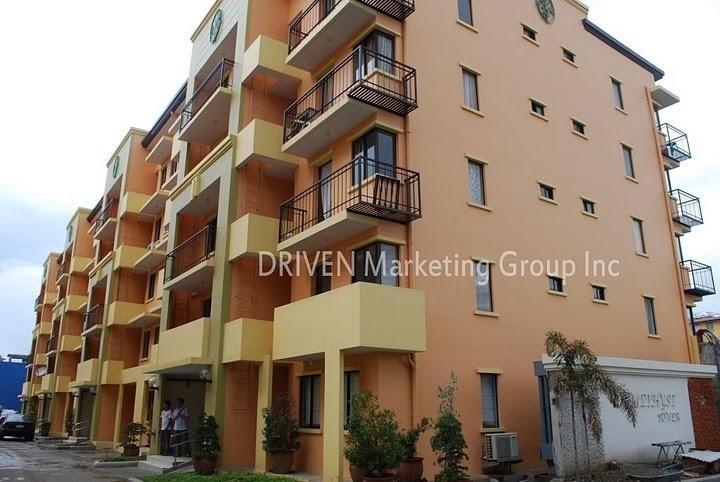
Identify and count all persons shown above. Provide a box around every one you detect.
[159,400,173,456]
[171,397,192,457]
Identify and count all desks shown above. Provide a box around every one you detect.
[651,440,691,467]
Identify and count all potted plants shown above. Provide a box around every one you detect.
[193,411,221,475]
[262,390,303,474]
[343,387,436,482]
[122,421,146,455]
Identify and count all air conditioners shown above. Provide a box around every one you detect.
[484,431,519,460]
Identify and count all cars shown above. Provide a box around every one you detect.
[0,409,35,442]
